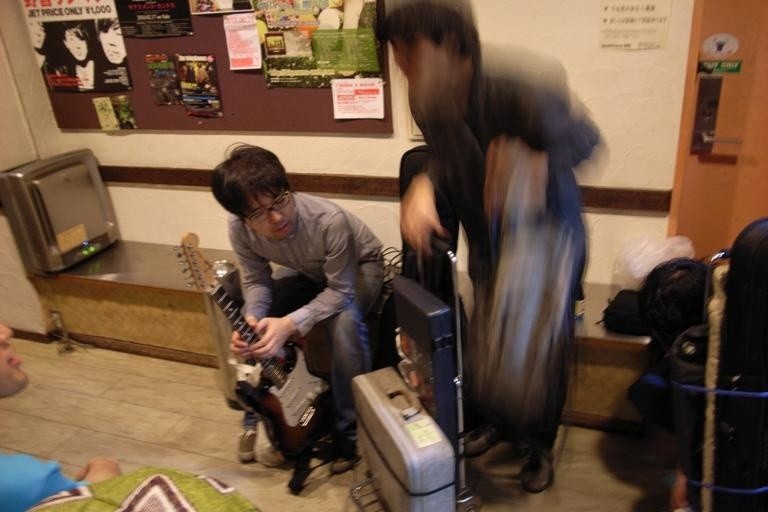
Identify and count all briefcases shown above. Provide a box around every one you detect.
[352,237,482,512]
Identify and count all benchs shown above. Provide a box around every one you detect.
[24,239,653,438]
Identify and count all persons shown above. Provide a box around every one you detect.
[94,15,127,68]
[30,20,48,68]
[208,140,384,476]
[371,0,610,494]
[62,22,97,92]
[0,321,122,511]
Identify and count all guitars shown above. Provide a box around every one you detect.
[173,234,334,470]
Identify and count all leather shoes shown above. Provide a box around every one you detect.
[464,425,496,456]
[520,455,553,493]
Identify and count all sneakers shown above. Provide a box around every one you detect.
[237,429,257,461]
[330,454,352,472]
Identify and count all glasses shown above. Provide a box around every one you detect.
[242,191,290,223]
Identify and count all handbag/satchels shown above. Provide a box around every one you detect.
[596,290,650,335]
